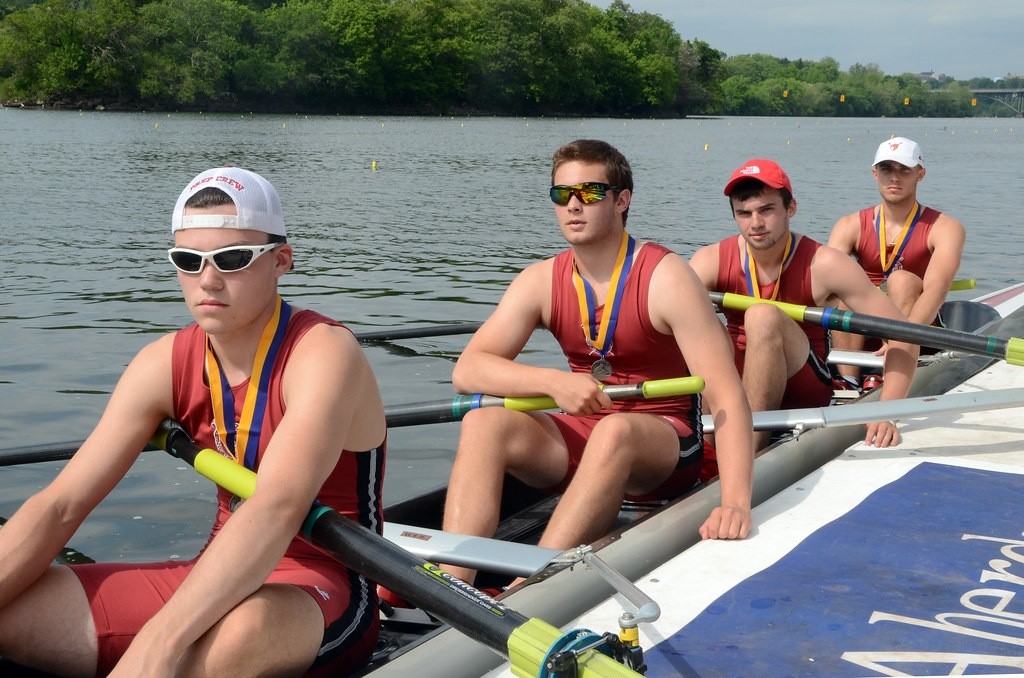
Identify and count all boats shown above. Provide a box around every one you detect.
[361,280,1024,678]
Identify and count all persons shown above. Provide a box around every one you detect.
[828,137,966,389]
[0,167,388,678]
[373,139,754,610]
[688,158,921,453]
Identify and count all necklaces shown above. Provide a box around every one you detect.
[886,229,899,246]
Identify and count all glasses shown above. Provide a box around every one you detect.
[549,181,624,207]
[167,242,285,275]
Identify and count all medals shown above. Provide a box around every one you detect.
[229,493,245,512]
[880,278,888,294]
[591,357,613,381]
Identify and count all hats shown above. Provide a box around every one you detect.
[723,158,793,198]
[870,135,925,171]
[170,166,287,238]
